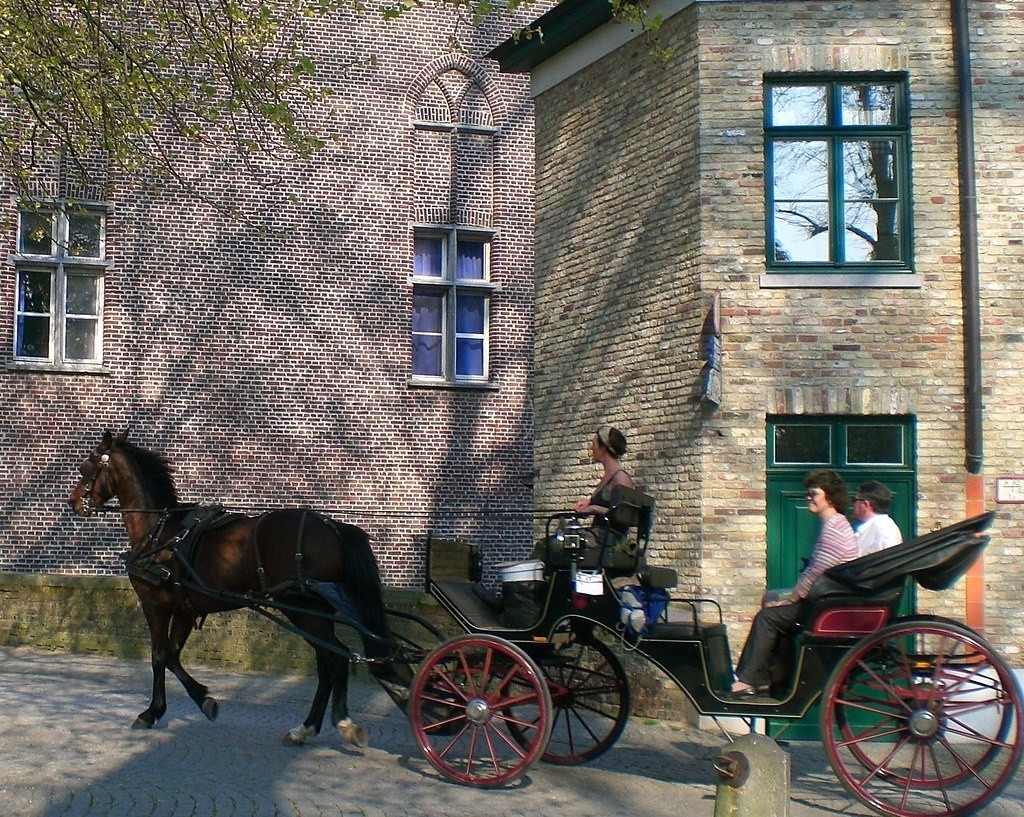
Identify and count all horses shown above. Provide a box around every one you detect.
[67,428,377,748]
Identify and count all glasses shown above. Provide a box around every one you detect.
[850,497,868,503]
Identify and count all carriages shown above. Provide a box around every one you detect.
[66,426,1023,817]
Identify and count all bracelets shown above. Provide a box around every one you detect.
[788,598,794,603]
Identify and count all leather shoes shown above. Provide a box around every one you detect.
[713,686,770,702]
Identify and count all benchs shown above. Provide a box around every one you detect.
[532,483,655,571]
[796,556,903,650]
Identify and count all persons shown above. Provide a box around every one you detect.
[730,469,858,694]
[851,481,904,559]
[472,425,634,612]
